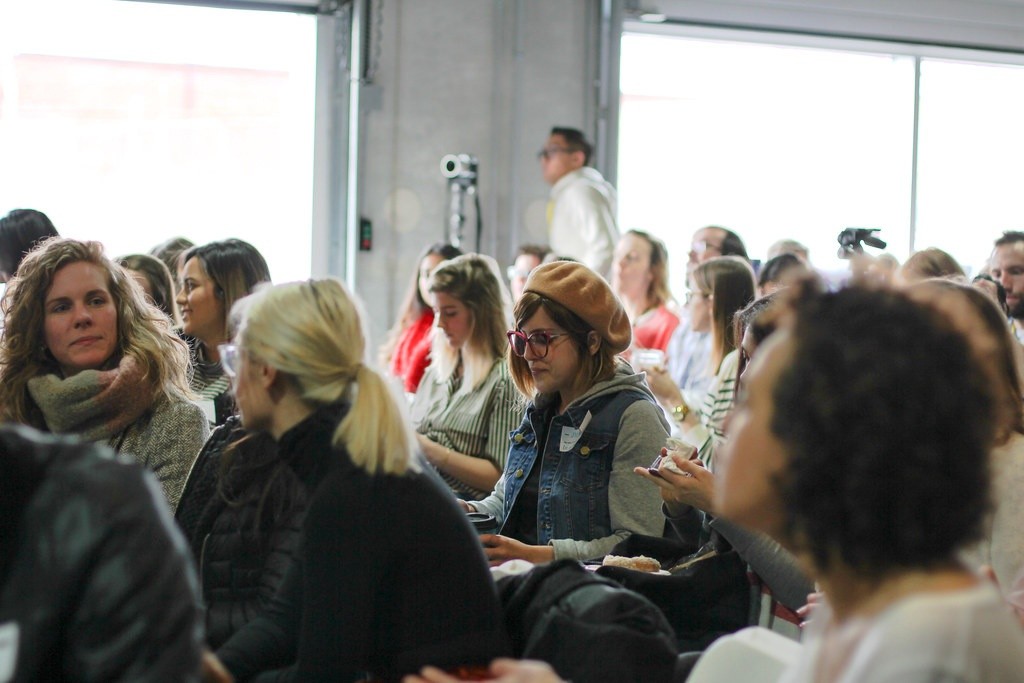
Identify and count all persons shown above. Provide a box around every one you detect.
[384,226,1024,683]
[536,126,619,277]
[0,208,496,683]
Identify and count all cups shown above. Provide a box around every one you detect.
[466,512,498,562]
[630,348,665,375]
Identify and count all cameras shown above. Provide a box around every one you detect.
[441,153,477,191]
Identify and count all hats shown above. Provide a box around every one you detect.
[522,262,632,355]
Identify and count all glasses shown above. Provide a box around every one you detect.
[507,330,575,358]
[537,145,577,160]
[218,345,246,376]
[688,239,722,257]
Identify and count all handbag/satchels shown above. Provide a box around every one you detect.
[492,558,678,683]
